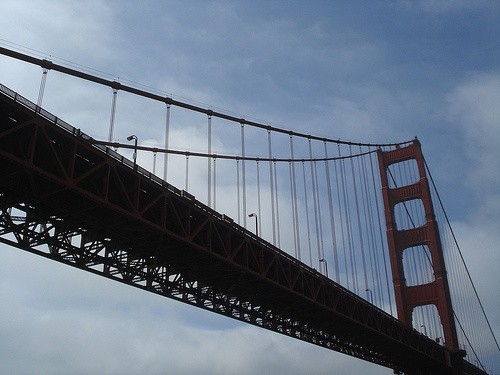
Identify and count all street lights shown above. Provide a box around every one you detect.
[365,289,373,305]
[127,135,138,165]
[248,213,258,236]
[319,259,328,278]
[419,323,495,375]
